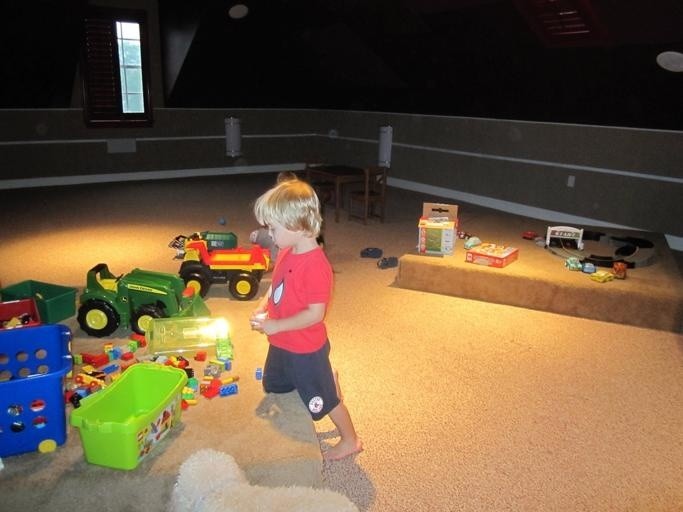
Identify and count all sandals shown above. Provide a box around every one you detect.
[362,248,382,258]
[376,257,398,268]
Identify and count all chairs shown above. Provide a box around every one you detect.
[304,154,390,225]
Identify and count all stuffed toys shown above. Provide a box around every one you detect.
[163,447,359,512]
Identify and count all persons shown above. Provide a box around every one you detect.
[249,170,325,262]
[248,181,364,463]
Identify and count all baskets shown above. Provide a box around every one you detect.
[0,325,74,458]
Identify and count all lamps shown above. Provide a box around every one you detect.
[224,115,242,160]
